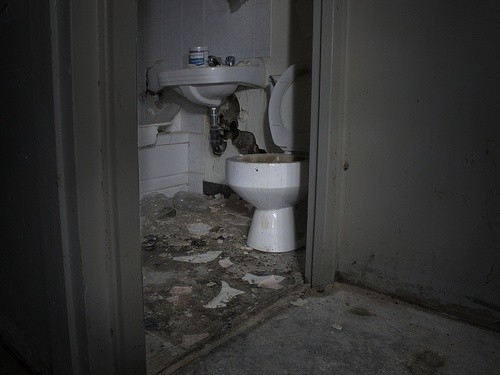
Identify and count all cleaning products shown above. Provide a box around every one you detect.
[188,46,208,67]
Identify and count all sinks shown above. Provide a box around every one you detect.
[156,64,266,109]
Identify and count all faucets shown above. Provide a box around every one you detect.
[208,54,224,66]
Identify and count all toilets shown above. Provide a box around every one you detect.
[225,60,312,253]
